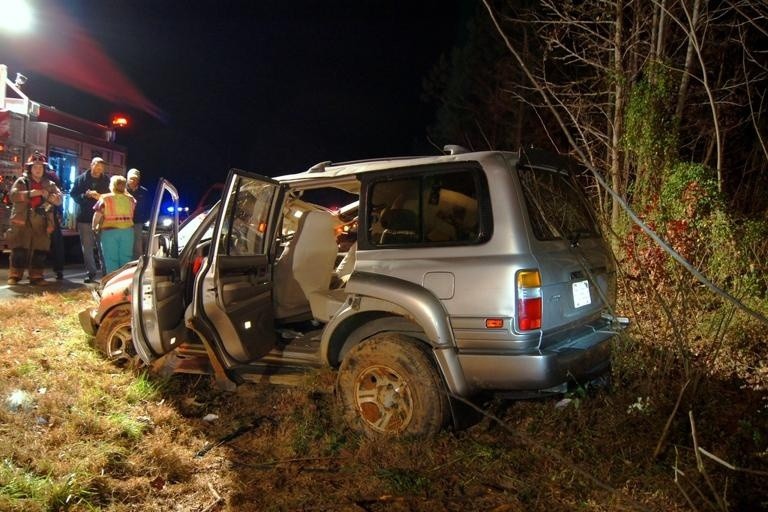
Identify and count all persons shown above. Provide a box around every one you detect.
[45,168,67,283]
[3,152,64,284]
[68,157,112,281]
[122,167,150,262]
[87,175,141,277]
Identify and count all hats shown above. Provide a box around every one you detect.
[23,150,49,168]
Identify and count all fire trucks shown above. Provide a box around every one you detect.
[0,64,130,270]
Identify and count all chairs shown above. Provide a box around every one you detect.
[272,208,421,322]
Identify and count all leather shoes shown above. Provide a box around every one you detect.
[8,277,17,285]
[55,272,63,280]
[84,272,96,283]
[31,278,48,287]
